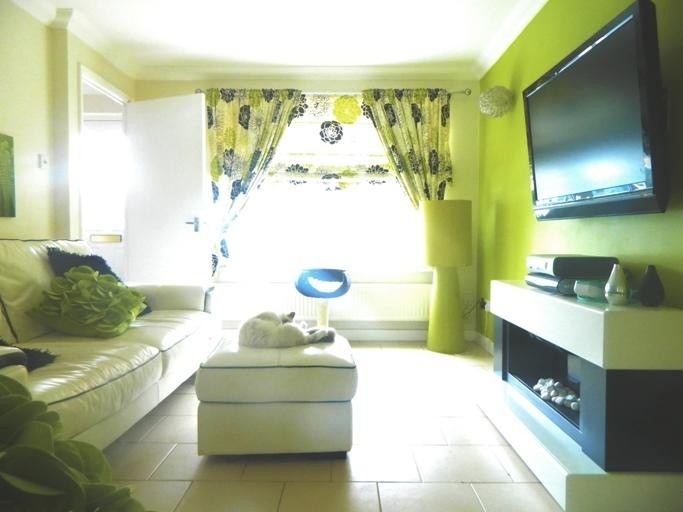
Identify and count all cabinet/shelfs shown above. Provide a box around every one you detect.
[478,275,681,512]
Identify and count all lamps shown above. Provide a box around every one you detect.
[420,198,475,354]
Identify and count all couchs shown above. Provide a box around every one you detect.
[0,235,222,456]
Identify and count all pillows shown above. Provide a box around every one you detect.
[22,264,149,341]
[45,244,150,316]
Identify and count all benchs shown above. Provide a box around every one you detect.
[190,318,357,460]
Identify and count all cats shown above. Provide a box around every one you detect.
[238,311,328,348]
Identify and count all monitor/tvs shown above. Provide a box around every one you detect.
[522,0,669,221]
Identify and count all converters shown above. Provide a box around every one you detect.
[480,301,485,308]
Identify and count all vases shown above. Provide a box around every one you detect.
[604,263,632,308]
[637,264,667,308]
